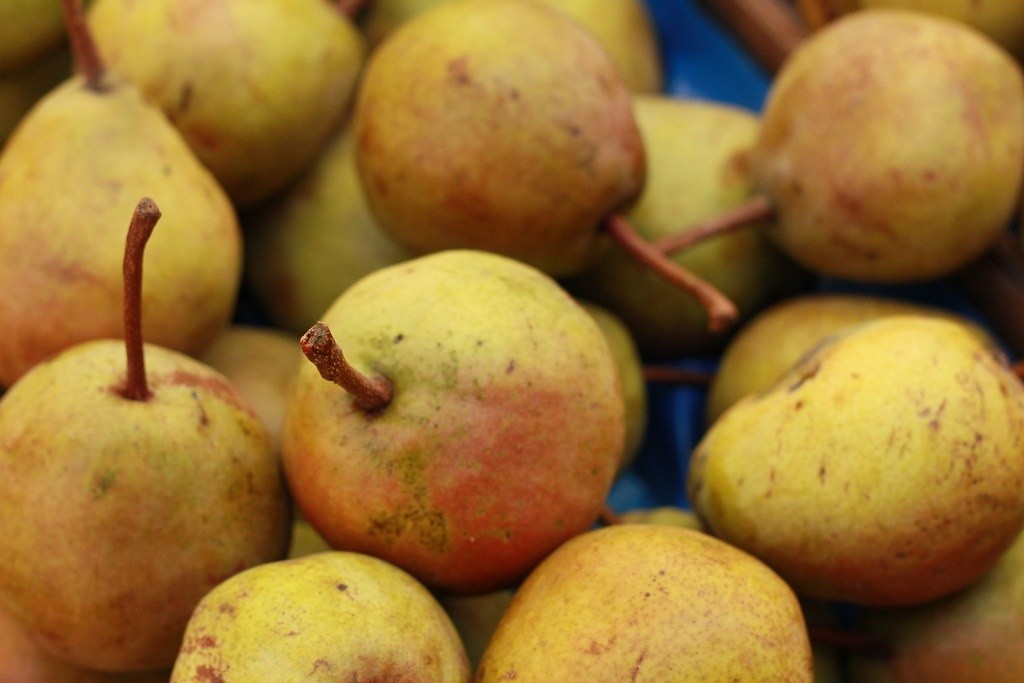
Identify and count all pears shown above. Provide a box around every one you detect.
[0,0,1024,682]
